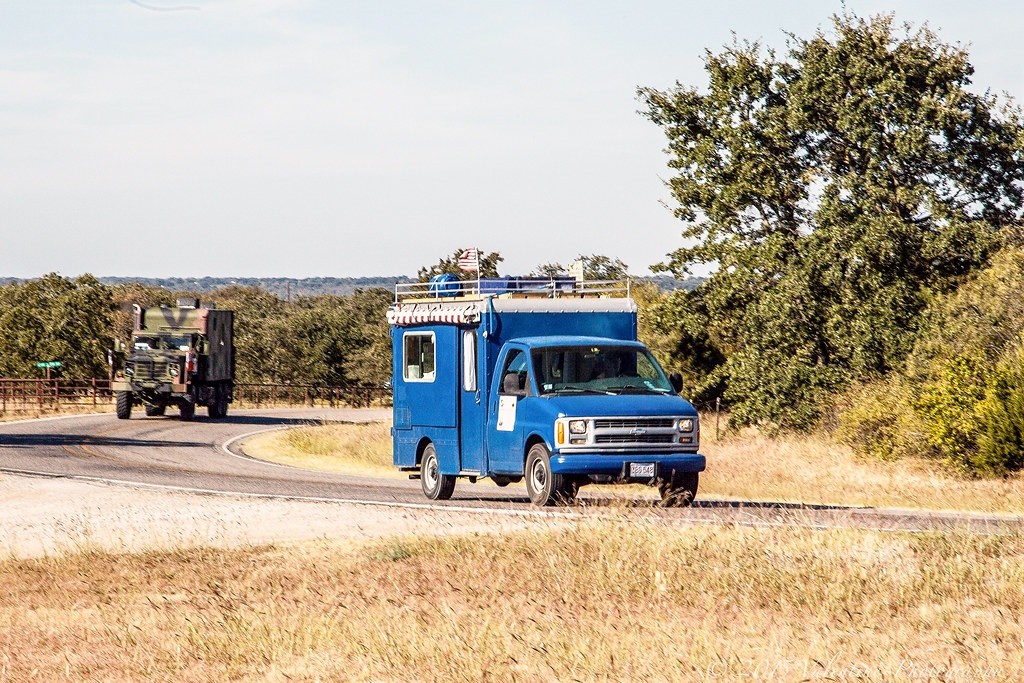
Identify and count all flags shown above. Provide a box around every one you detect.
[569,260,583,277]
[457,248,479,271]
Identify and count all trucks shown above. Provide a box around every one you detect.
[108,297,237,420]
[386,247,706,508]
